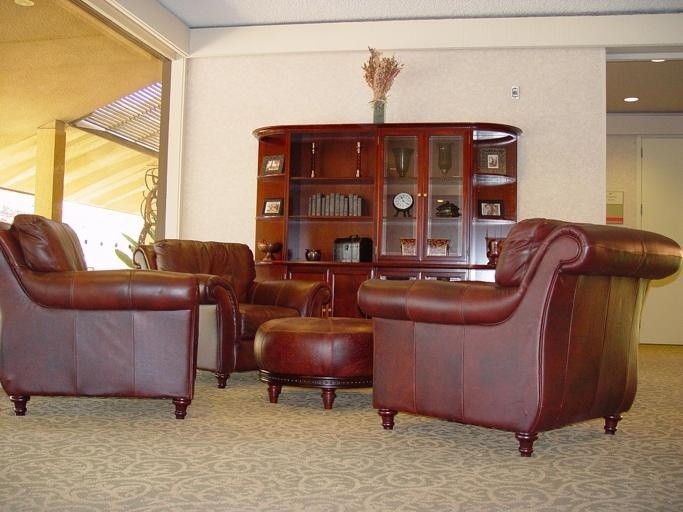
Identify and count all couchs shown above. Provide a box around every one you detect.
[0,218,210,420]
[130,234,336,389]
[354,217,680,453]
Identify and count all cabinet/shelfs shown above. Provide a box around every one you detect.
[252,122,523,319]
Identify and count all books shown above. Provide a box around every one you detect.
[308,192,362,216]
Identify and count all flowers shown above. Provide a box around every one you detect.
[363,47,405,98]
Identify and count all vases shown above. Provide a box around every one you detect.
[373,98,384,124]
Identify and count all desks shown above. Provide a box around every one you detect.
[248,315,372,410]
